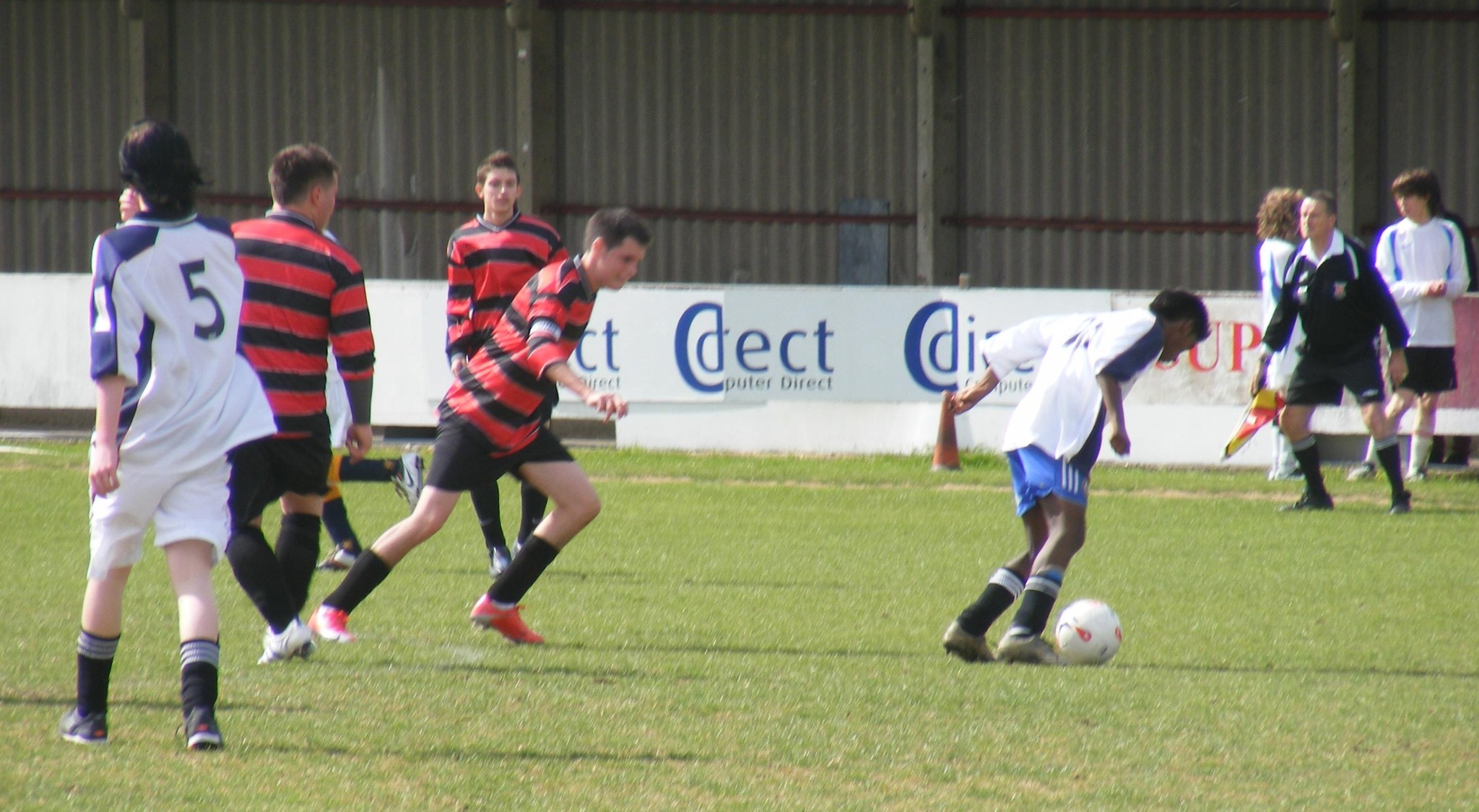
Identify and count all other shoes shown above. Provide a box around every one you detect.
[1405,467,1428,482]
[1284,469,1306,481]
[1348,461,1377,481]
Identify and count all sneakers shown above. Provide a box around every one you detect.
[60,706,107,745]
[994,633,1064,666]
[175,707,222,751]
[1390,501,1411,515]
[469,593,544,643]
[512,539,523,555]
[488,547,512,577]
[942,621,994,662]
[307,605,354,643]
[315,544,357,570]
[257,617,312,665]
[391,454,425,515]
[1280,490,1334,510]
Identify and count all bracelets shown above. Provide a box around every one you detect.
[1258,358,1266,364]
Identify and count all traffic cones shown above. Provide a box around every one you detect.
[930,388,963,471]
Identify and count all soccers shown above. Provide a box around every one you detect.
[1053,598,1122,667]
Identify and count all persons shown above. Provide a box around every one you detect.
[306,208,654,644]
[228,144,424,664]
[444,150,571,579]
[946,291,1211,659]
[1251,191,1412,516]
[57,117,279,750]
[1256,187,1324,481]
[1346,169,1475,482]
